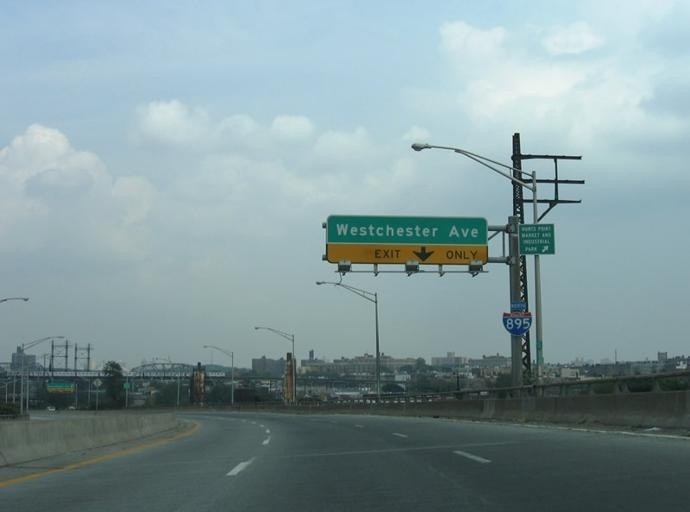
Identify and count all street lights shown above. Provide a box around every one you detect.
[0,295,30,305]
[254,327,296,407]
[203,345,234,410]
[315,280,382,408]
[412,144,547,398]
[19,334,64,416]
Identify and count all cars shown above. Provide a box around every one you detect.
[68,405,75,412]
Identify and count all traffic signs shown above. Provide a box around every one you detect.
[517,224,554,255]
[322,215,490,267]
